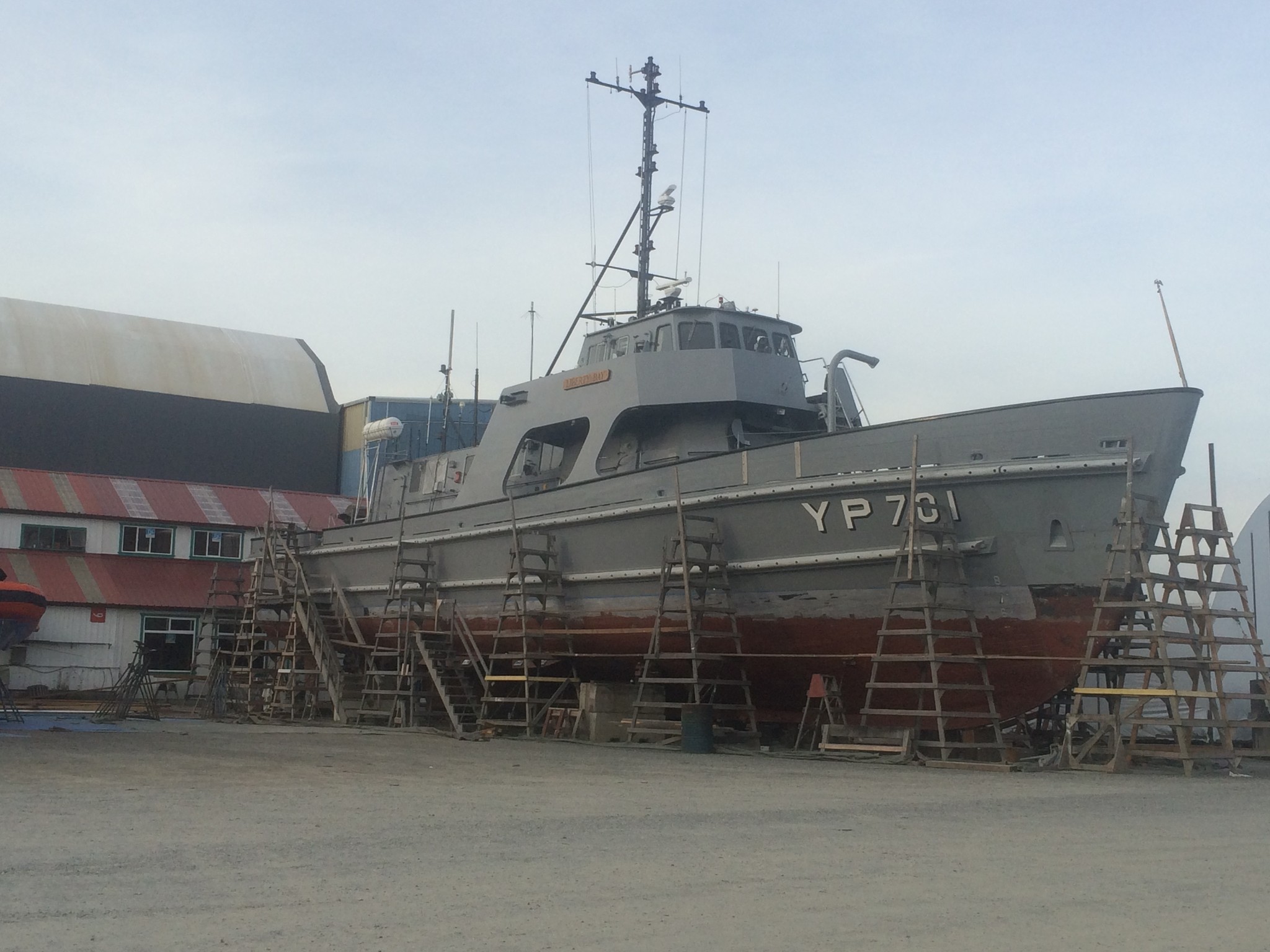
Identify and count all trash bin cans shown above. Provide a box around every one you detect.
[680,702,714,754]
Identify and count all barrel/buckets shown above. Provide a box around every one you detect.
[681,704,714,754]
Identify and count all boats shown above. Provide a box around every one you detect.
[236,57,1208,739]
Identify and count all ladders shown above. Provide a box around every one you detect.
[626,513,762,751]
[860,501,1011,764]
[1056,492,1235,778]
[184,562,252,708]
[792,673,856,753]
[221,557,292,713]
[355,541,453,730]
[270,607,335,723]
[1111,583,1180,745]
[475,528,581,740]
[1035,677,1089,744]
[1125,502,1270,775]
